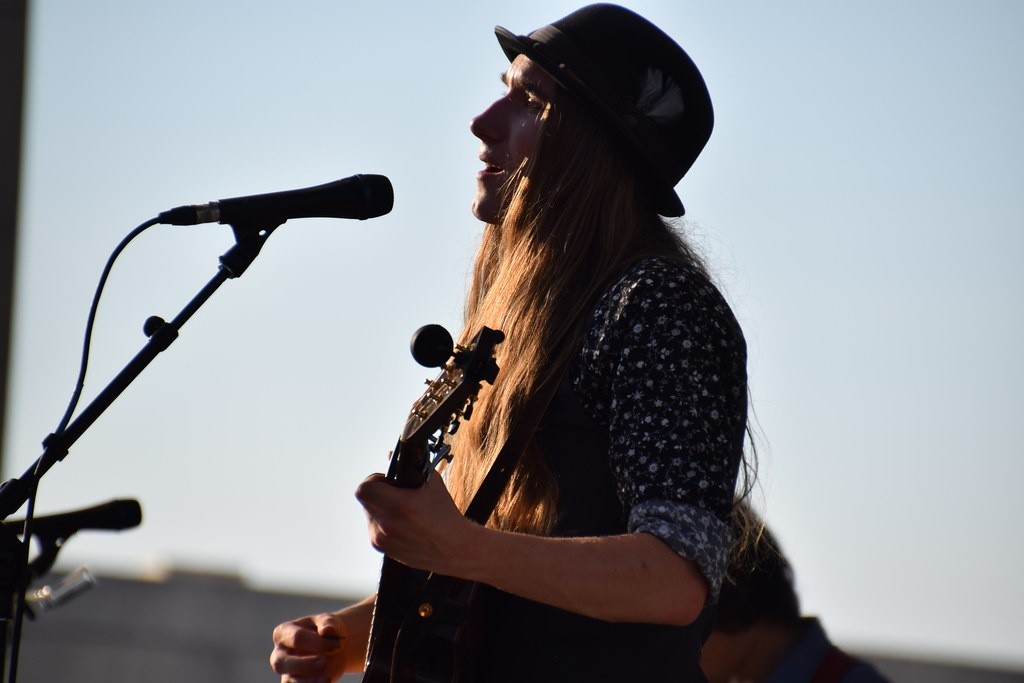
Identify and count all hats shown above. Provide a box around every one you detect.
[494,2,715,218]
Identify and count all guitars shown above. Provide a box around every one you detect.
[364,323,508,683]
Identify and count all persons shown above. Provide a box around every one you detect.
[702,496,889,683]
[270,2,760,683]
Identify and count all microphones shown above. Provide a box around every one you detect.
[158,174,394,225]
[3,499,142,536]
